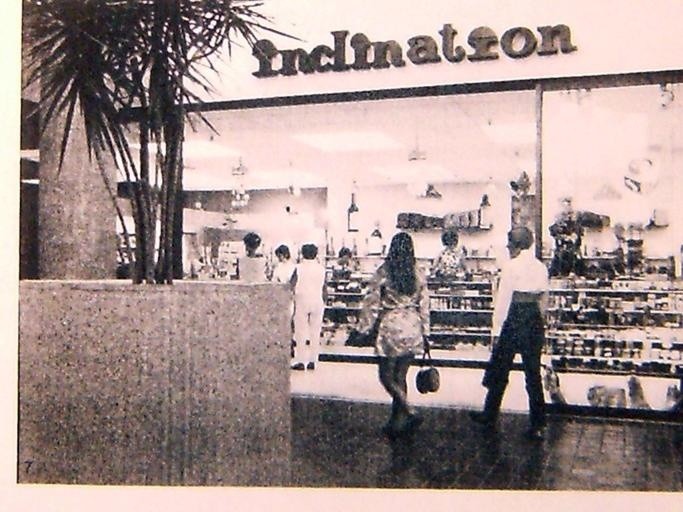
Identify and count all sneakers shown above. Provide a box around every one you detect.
[468,410,498,426]
[376,412,426,439]
[523,429,545,440]
[290,363,313,370]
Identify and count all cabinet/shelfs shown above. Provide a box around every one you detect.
[323,255,682,375]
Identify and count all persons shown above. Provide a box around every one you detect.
[471,226,548,441]
[357,231,432,437]
[269,245,294,284]
[334,244,354,276]
[549,196,587,278]
[431,229,469,285]
[287,244,330,372]
[233,231,268,283]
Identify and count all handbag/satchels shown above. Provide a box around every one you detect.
[416,368,440,394]
